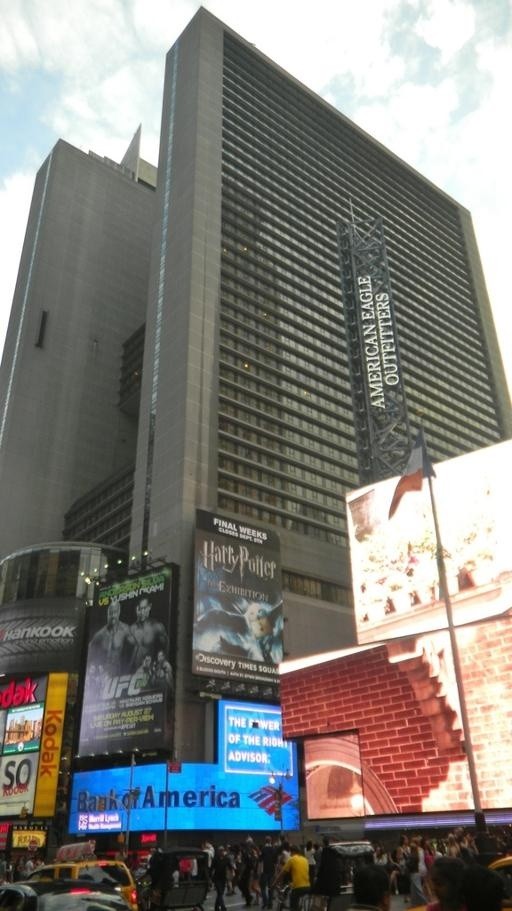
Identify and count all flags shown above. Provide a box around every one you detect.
[388,427,436,521]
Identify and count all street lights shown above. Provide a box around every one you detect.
[98,795,139,856]
[269,769,294,838]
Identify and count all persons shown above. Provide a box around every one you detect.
[114,842,157,869]
[97,663,110,691]
[135,852,176,907]
[428,857,504,911]
[221,603,284,665]
[373,826,511,904]
[88,601,139,679]
[152,650,173,693]
[130,655,154,695]
[361,835,369,841]
[85,664,99,702]
[347,865,390,911]
[130,595,167,664]
[355,855,364,864]
[201,834,347,911]
[13,855,45,883]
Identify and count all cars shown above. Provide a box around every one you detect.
[0,860,140,911]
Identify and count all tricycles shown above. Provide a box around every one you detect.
[268,840,377,910]
[138,846,209,911]
